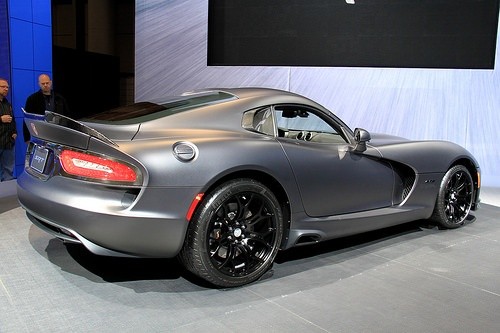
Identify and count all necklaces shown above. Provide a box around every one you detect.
[47,97,51,106]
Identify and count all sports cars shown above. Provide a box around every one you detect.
[14,84,482,289]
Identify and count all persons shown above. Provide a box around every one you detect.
[0,79,18,183]
[23,73,66,144]
